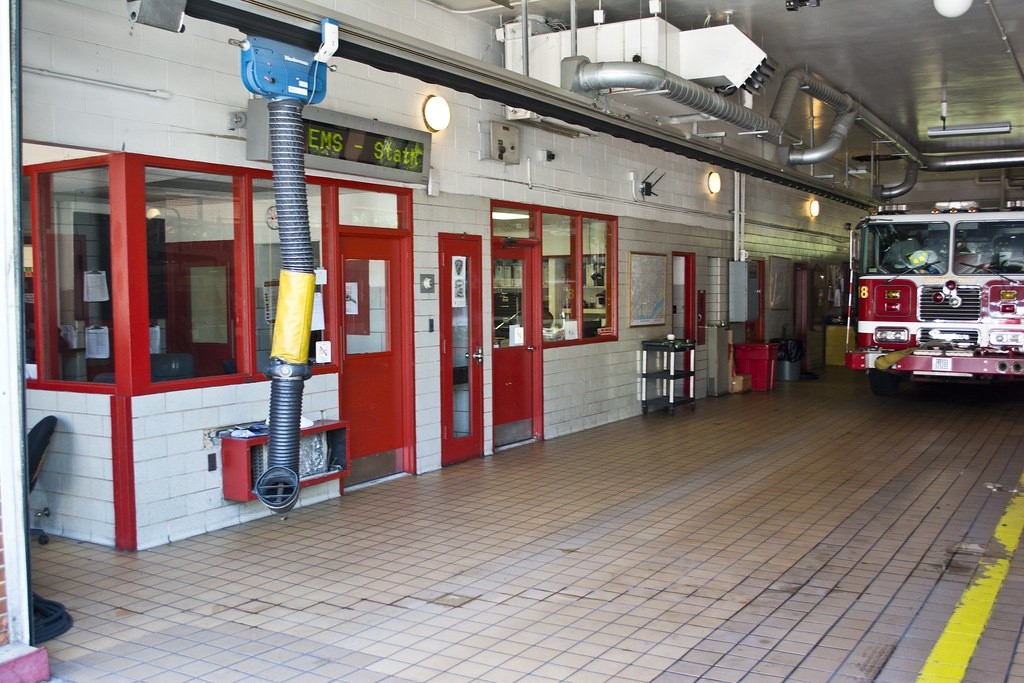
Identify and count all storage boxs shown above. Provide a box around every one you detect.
[734,374,751,393]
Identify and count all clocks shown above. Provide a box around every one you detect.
[266,206,279,230]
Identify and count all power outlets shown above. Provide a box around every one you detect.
[630,171,638,181]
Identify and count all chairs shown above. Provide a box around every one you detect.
[27,415,58,544]
[884,240,920,261]
[995,234,1024,268]
[150,352,195,381]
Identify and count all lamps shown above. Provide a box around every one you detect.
[810,200,820,217]
[708,172,721,194]
[927,123,1011,138]
[423,95,451,132]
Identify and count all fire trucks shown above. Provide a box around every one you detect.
[845,203,1024,398]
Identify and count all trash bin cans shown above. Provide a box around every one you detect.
[769,337,806,383]
[734,341,779,392]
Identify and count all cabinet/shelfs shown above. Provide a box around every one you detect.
[641,338,697,416]
[826,325,857,366]
[494,253,606,344]
